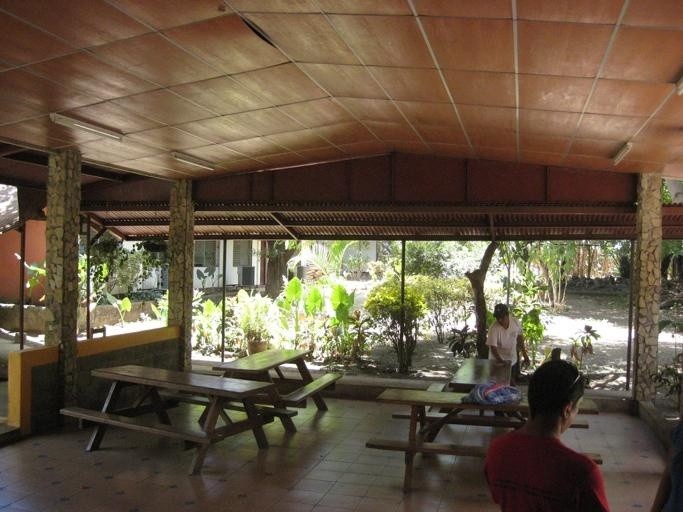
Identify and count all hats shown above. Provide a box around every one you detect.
[493,304,508,317]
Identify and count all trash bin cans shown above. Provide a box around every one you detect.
[242,267,254,285]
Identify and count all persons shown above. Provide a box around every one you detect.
[649,453,683,512]
[485,304,530,418]
[484,359,611,512]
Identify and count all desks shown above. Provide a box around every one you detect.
[448,358,512,388]
[86,350,328,476]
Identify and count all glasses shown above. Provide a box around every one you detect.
[568,368,581,388]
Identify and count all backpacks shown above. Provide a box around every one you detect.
[461,380,522,406]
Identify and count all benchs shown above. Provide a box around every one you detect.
[60,368,343,477]
[366,383,603,496]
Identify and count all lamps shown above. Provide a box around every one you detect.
[169,151,215,171]
[674,76,683,96]
[48,112,123,143]
[613,142,633,168]
